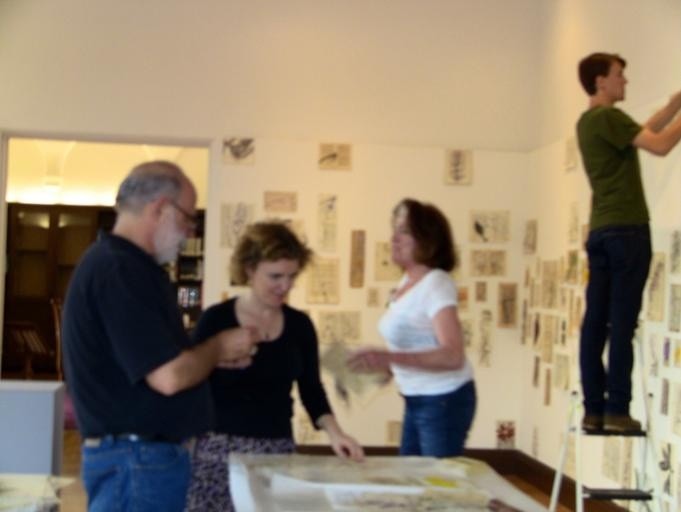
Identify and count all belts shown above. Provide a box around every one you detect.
[115,433,166,441]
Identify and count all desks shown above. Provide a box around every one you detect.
[0,472,76,512]
[227,451,551,511]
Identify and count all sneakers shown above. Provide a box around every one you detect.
[605,414,641,431]
[582,416,604,431]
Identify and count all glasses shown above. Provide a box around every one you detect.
[153,196,197,223]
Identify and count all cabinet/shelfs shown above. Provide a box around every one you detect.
[162,208,205,328]
[0,203,112,379]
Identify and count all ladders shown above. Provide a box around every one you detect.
[548,325,664,512]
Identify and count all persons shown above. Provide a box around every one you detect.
[573,52,681,436]
[181,217,366,512]
[58,159,261,511]
[342,197,478,460]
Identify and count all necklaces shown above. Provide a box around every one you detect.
[246,310,284,340]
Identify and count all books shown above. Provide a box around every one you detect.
[175,236,203,327]
[267,459,427,493]
[325,480,528,511]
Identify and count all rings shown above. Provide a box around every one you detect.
[250,345,257,354]
